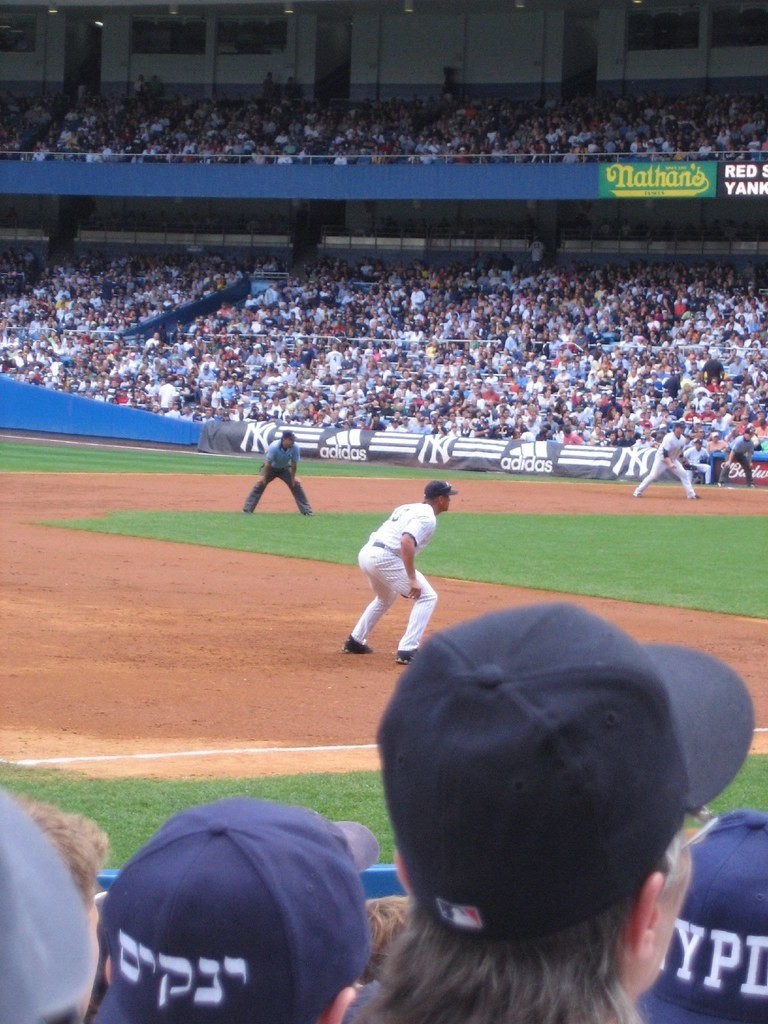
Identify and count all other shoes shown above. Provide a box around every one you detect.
[305,511,314,515]
[242,509,253,514]
[342,634,372,654]
[691,495,699,499]
[633,492,643,497]
[717,483,725,487]
[396,649,419,665]
[750,484,758,488]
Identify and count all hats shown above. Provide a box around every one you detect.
[694,439,703,444]
[93,795,381,1024]
[424,480,458,498]
[283,430,297,440]
[461,366,466,370]
[376,603,755,939]
[632,809,768,1024]
[745,428,754,435]
[253,344,262,348]
[674,422,687,428]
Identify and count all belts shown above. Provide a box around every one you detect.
[373,542,385,548]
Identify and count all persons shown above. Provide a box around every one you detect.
[683,438,715,485]
[632,421,703,499]
[328,601,757,1024]
[242,430,314,516]
[717,428,755,488]
[0,788,768,1024]
[0,70,768,461]
[341,479,459,664]
